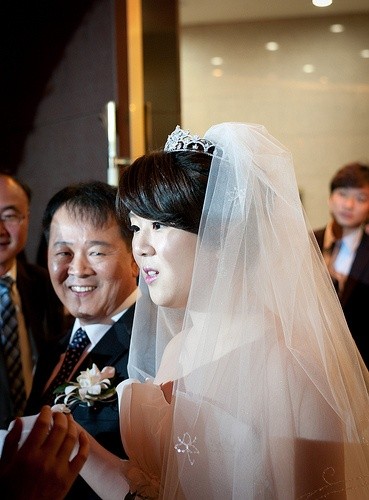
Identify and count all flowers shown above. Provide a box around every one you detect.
[66,364,116,401]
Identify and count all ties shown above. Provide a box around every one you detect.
[0,275,27,432]
[37,327,91,419]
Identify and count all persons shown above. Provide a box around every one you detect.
[115,120,369,500]
[312,161,369,374]
[0,175,141,500]
